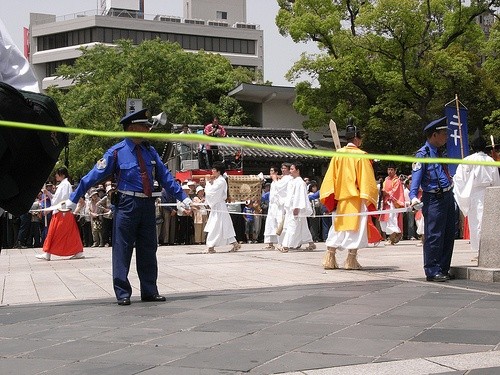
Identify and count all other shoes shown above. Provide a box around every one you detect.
[70,252,85,259]
[346,249,362,269]
[390,232,402,245]
[202,247,216,254]
[35,253,50,260]
[324,246,339,268]
[262,246,275,250]
[306,244,316,250]
[281,248,288,253]
[230,244,241,252]
[84,242,113,247]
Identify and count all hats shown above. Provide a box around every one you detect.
[119,108,153,125]
[182,185,190,189]
[387,161,396,167]
[46,181,53,185]
[196,186,204,194]
[424,116,452,132]
[89,188,98,198]
[345,117,362,139]
[471,128,486,150]
[187,181,196,186]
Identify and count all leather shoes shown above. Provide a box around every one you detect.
[118,298,130,305]
[142,295,166,301]
[447,275,455,280]
[427,274,446,281]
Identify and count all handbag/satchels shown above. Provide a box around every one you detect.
[0,81,70,218]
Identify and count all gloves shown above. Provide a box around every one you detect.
[183,197,193,209]
[412,198,424,210]
[65,199,77,211]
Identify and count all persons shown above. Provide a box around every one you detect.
[201,161,241,254]
[319,125,379,270]
[375,168,422,241]
[0,179,117,248]
[151,170,332,247]
[378,163,405,246]
[452,135,500,263]
[264,166,281,249]
[274,163,302,250]
[277,161,316,253]
[36,167,86,261]
[61,108,206,304]
[178,116,227,172]
[409,117,456,282]
[0,17,69,253]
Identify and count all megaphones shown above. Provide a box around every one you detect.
[152,112,167,125]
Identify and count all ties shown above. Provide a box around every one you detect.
[137,146,151,197]
[443,164,452,183]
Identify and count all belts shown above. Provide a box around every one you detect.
[428,184,454,193]
[118,190,148,197]
